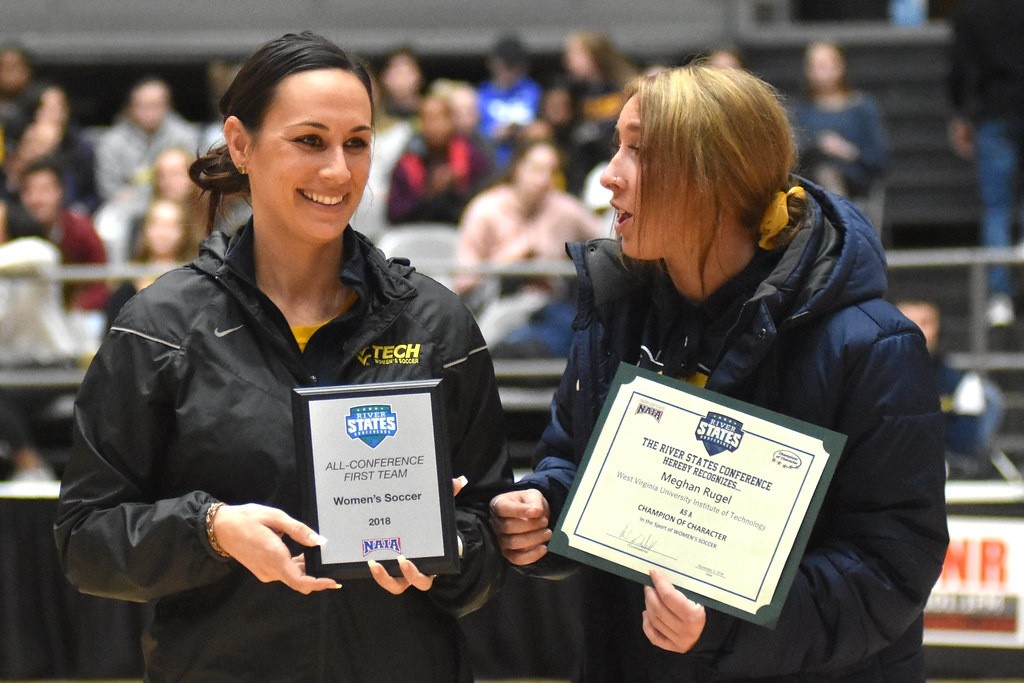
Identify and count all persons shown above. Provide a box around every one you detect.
[0,40,1024,494]
[55,30,517,683]
[488,64,952,683]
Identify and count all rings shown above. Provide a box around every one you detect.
[492,498,500,513]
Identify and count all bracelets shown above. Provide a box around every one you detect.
[205,502,231,558]
